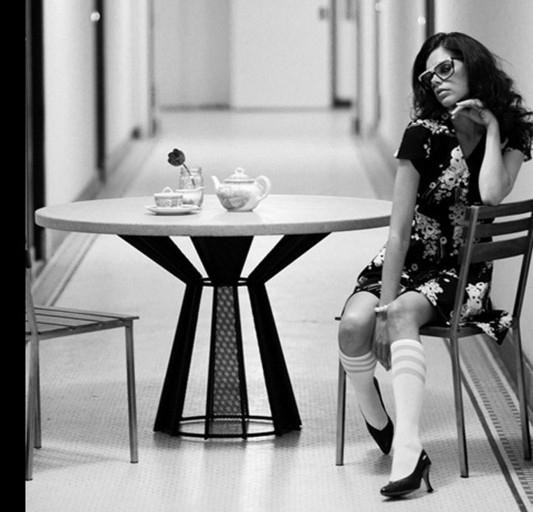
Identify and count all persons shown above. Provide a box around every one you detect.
[338,30,533,497]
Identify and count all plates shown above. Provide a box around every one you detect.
[145,203,200,215]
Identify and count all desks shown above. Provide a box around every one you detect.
[34,194,393,440]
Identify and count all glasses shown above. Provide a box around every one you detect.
[417,57,465,87]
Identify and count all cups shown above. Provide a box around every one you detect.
[153,187,183,208]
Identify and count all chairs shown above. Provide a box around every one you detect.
[334,198,533,478]
[25,245,140,481]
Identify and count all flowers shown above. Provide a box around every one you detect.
[167,148,196,187]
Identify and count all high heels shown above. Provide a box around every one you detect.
[381,450,433,497]
[361,376,392,454]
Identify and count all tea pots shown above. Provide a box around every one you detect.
[211,167,272,212]
